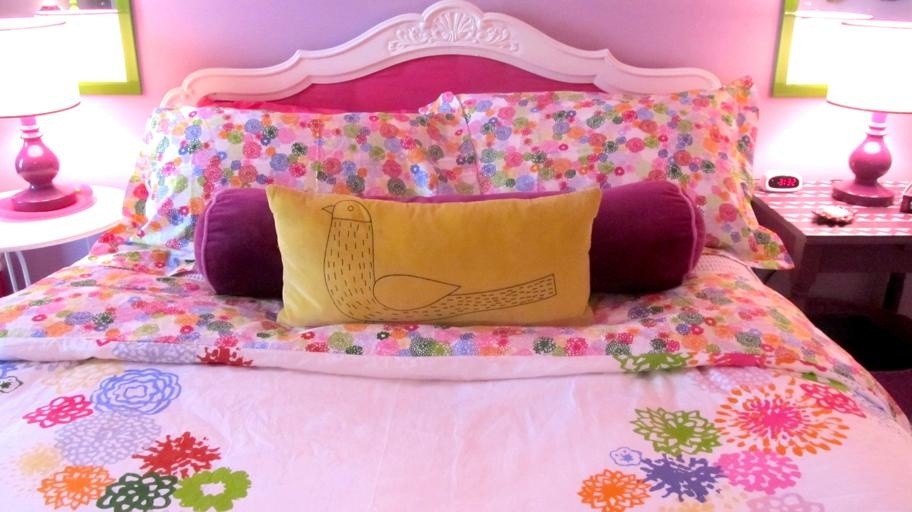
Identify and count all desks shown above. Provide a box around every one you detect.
[0,184,130,297]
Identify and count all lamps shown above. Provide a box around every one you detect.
[827,19,912,206]
[0,17,84,213]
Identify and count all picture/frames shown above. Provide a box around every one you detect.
[769,0,911,98]
[0,0,144,97]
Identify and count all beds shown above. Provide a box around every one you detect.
[0,2,912,512]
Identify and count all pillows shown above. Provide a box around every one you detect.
[189,181,706,297]
[133,92,483,260]
[422,73,797,272]
[267,183,599,328]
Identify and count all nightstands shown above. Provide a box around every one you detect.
[742,168,911,376]
